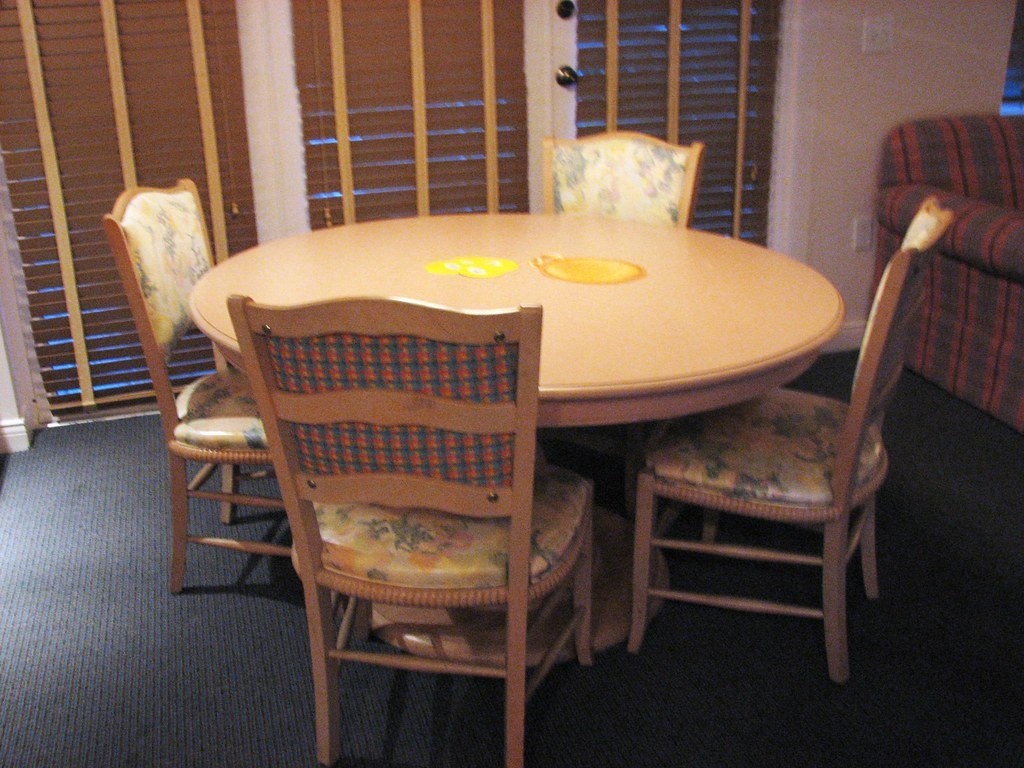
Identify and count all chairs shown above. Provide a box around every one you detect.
[104,177,292,594]
[628,194,957,687]
[227,293,593,768]
[540,129,709,511]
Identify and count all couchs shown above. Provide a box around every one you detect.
[876,115,1024,433]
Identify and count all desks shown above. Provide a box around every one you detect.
[189,214,844,670]
